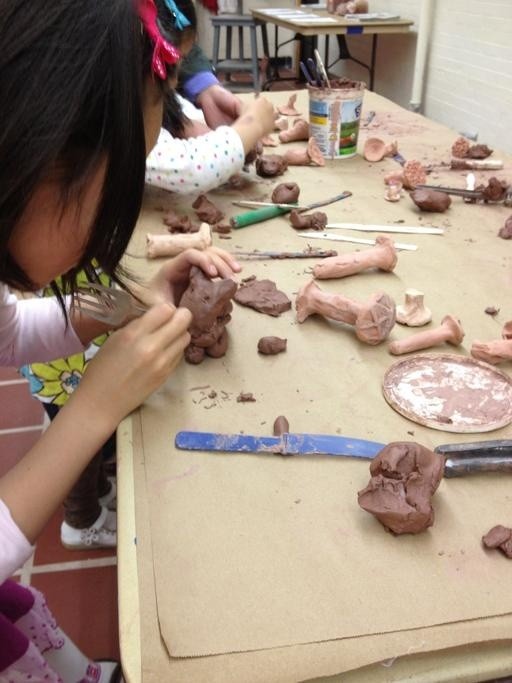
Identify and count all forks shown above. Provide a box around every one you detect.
[70,280,144,328]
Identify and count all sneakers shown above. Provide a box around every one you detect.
[92,656,125,683]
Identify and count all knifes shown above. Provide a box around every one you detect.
[174,431,387,460]
[223,250,336,259]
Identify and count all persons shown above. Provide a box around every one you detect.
[7,1,275,551]
[1,2,242,681]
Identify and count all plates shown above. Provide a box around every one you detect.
[382,351,511,432]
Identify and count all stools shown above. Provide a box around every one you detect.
[208,14,270,93]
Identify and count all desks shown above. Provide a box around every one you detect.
[114,88,512,683]
[249,6,414,91]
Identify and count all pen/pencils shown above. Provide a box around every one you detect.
[232,199,314,209]
[299,51,331,87]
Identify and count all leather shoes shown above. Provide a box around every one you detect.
[101,477,117,510]
[59,506,116,550]
